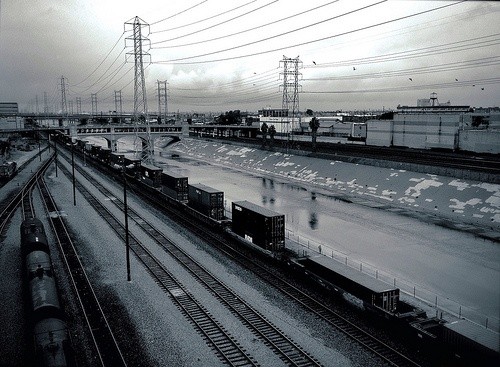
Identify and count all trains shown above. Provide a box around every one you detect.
[20,217,78,367]
[0,160,18,181]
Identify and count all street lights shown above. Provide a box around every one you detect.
[113,164,135,281]
[50,133,59,177]
[66,142,79,206]
